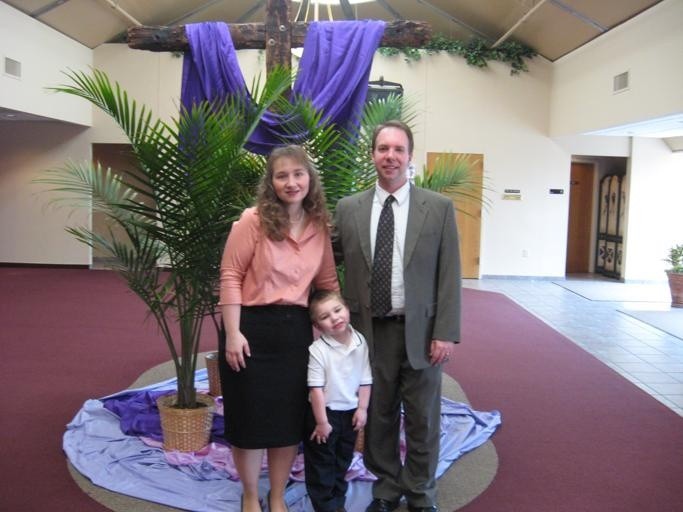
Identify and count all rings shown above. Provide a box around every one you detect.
[446,352,450,357]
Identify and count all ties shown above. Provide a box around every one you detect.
[371,196,395,318]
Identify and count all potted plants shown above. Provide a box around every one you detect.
[663,246,683,308]
[27,90,266,455]
[44,61,302,397]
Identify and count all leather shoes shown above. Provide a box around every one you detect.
[366,498,395,512]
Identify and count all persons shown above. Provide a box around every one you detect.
[328,118,462,512]
[303,289,373,511]
[217,143,343,512]
